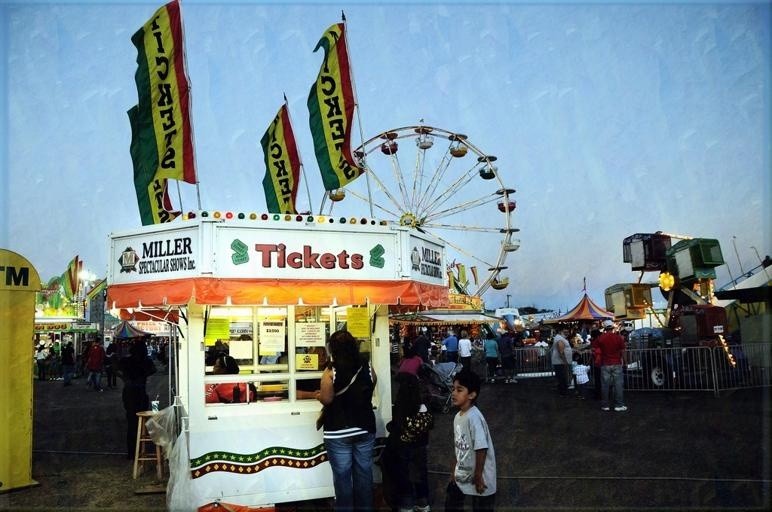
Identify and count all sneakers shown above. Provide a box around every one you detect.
[413,498,431,512]
[383,495,414,512]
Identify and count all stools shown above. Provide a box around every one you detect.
[132,411,166,481]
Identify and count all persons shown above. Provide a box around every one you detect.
[313,331,377,511]
[374,384,434,510]
[445,370,496,511]
[35,332,179,464]
[207,320,629,413]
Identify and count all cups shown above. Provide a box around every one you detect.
[151,400,160,413]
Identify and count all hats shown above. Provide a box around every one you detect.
[602,320,613,328]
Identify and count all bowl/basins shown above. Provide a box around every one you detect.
[262,397,282,402]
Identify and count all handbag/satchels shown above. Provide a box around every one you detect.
[316,406,325,430]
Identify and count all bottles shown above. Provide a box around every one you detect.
[419,404,428,413]
